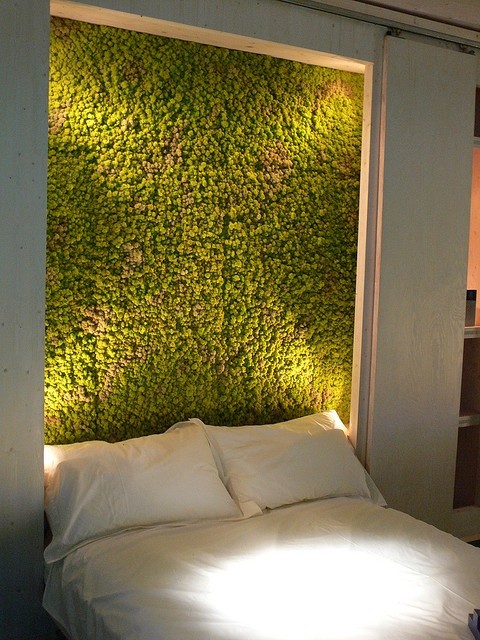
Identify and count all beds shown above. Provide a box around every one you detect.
[48,497,480,640]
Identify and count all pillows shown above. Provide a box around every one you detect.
[203,409,388,514]
[44,421,248,563]
